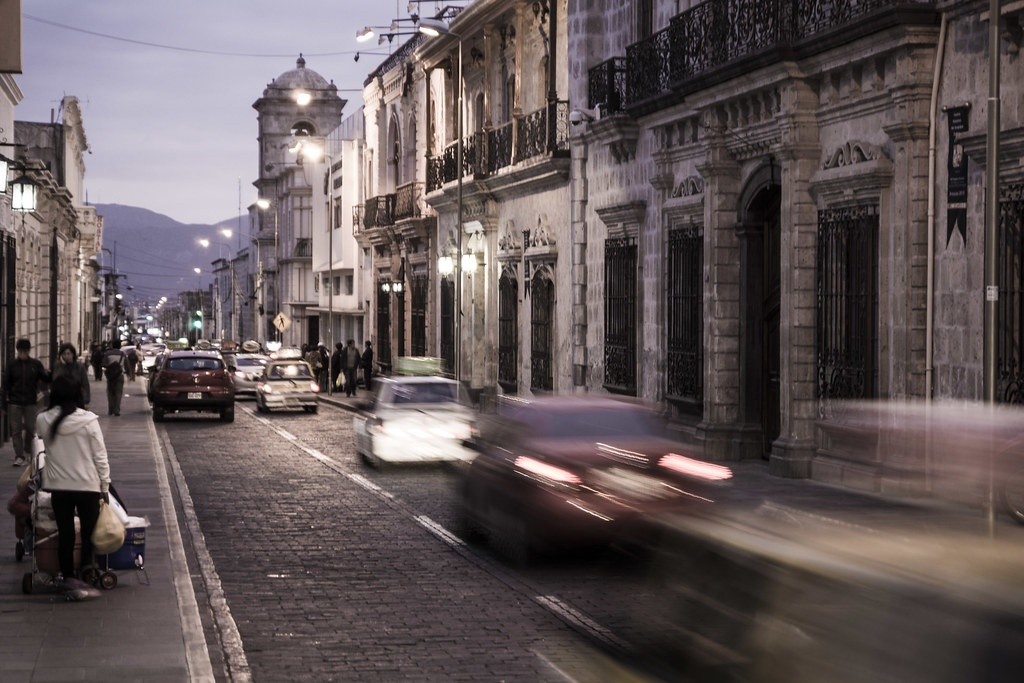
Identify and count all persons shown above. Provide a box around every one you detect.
[302,339,373,396]
[36,371,110,599]
[236,341,265,353]
[4,338,91,466]
[90,339,144,415]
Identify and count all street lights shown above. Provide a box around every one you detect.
[302,143,334,397]
[255,199,279,341]
[198,239,234,340]
[414,18,464,380]
[194,267,221,340]
[223,227,260,342]
[160,297,182,338]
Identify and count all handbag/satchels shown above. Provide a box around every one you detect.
[341,372,346,385]
[91,503,125,555]
[336,373,342,387]
[105,361,122,381]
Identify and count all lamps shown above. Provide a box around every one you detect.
[0,127,47,212]
[532,0,540,19]
[380,279,406,298]
[437,248,478,276]
[471,37,477,63]
[354,0,459,63]
[475,229,486,240]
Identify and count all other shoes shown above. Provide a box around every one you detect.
[13,457,23,466]
[65,587,80,601]
[115,413,120,416]
[108,412,112,415]
[26,453,31,463]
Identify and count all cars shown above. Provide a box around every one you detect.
[252,357,322,415]
[457,393,739,566]
[351,376,481,472]
[125,327,302,396]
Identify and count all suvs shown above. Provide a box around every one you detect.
[147,348,237,424]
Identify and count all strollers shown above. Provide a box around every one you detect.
[7,450,119,597]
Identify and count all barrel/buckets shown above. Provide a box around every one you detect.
[108,527,145,570]
